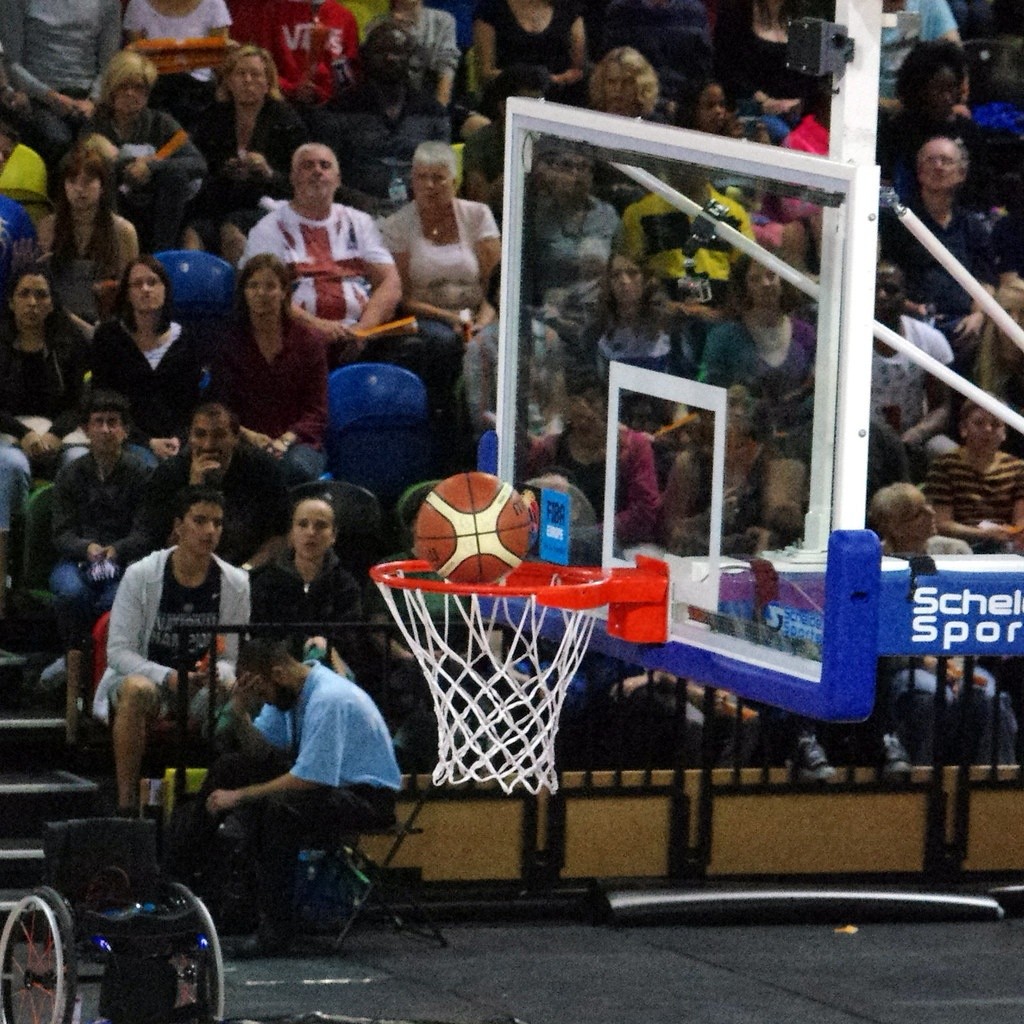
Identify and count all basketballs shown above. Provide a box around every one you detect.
[415,471,531,586]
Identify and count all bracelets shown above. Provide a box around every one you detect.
[277,434,294,448]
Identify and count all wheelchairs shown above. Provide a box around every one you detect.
[0,815,226,1024]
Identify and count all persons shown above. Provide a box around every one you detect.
[0,0,1024,818]
[181,634,404,960]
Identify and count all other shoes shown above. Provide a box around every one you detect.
[880,734,909,773]
[793,737,836,781]
[235,920,292,958]
[39,655,88,691]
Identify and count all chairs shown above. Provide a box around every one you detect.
[0,0,1024,765]
[276,768,448,951]
